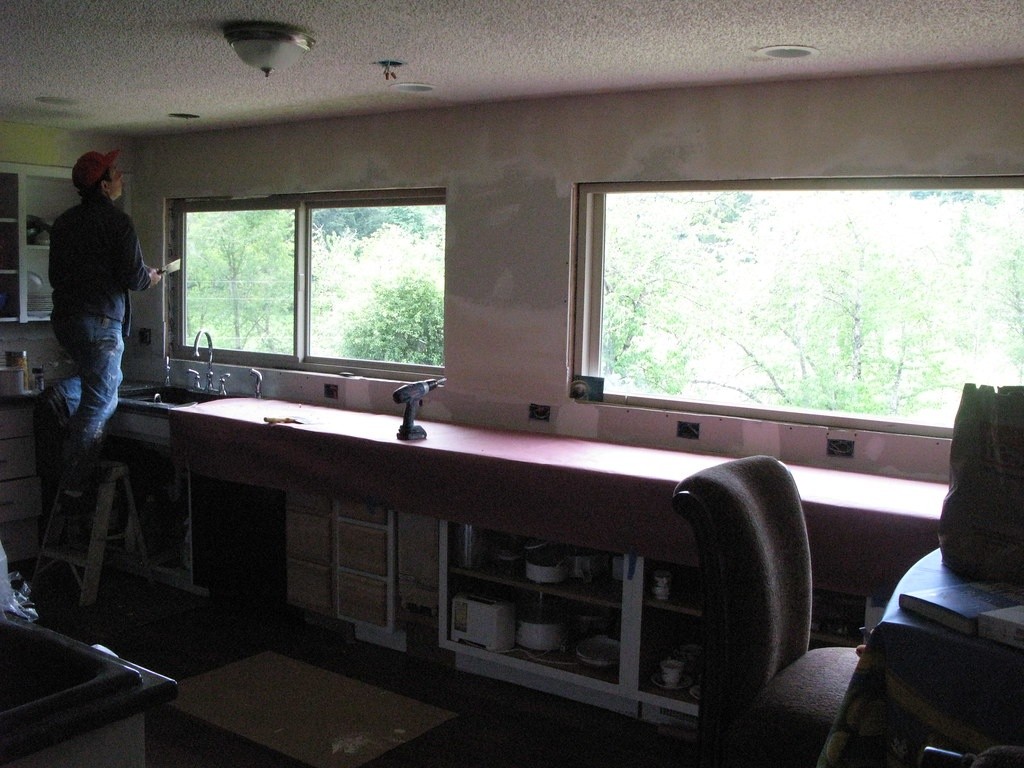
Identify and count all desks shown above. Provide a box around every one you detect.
[814,546,1024,768]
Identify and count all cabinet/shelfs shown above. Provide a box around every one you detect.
[0,394,43,564]
[436,506,706,734]
[1,159,74,323]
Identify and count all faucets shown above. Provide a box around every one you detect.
[192,329,214,388]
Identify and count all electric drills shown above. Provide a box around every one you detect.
[393,376,447,442]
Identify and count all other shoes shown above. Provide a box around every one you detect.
[57,487,121,517]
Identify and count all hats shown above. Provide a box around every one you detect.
[72,149,121,191]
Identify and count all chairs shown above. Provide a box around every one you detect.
[671,457,869,767]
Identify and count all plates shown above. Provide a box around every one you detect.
[651,671,693,688]
[690,685,700,700]
[577,638,621,666]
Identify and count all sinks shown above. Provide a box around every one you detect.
[118,386,236,405]
[1,618,138,725]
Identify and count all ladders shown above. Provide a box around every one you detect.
[26,460,159,608]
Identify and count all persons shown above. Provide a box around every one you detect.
[37,148,165,506]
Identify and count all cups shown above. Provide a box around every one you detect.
[456,524,484,568]
[660,659,684,683]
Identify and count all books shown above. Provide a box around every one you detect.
[898,579,1024,632]
[977,605,1024,646]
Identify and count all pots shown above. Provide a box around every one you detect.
[514,607,567,651]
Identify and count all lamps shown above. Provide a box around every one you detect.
[222,21,317,77]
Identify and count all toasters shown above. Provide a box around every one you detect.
[450,592,515,652]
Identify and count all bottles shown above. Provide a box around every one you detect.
[654,571,672,601]
